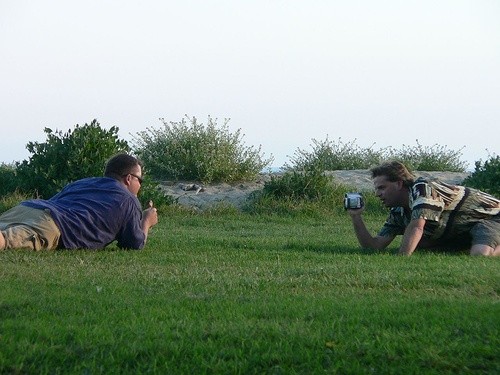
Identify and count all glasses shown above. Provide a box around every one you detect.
[123,173,143,184]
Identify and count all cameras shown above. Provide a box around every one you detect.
[344,193,363,209]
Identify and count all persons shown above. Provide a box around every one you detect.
[0,153,159,252]
[345,160,500,258]
